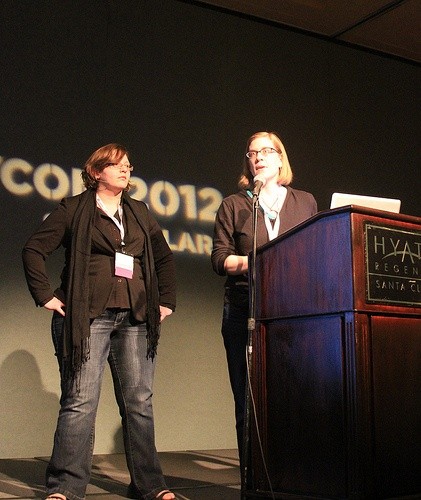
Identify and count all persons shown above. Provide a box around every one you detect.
[23,143,177,499]
[210,132,317,499]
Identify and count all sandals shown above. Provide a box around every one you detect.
[155,489,176,500]
[45,494,67,500]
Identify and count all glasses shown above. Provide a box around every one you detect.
[245,147,282,159]
[104,162,134,172]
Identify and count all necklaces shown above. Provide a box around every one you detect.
[259,186,281,222]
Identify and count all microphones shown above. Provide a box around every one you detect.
[252,173,266,197]
